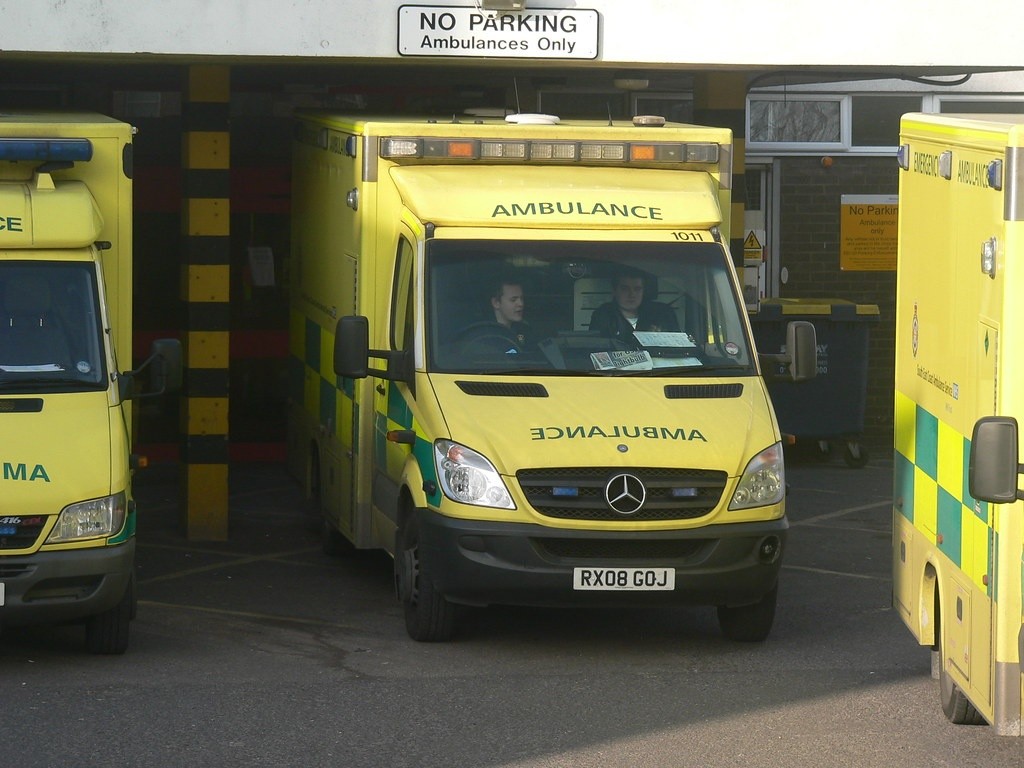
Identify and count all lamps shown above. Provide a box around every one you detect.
[613,70,650,90]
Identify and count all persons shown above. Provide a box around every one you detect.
[465,276,530,352]
[590,265,678,341]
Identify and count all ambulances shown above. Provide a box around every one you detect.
[283,111,788,648]
[0,109,145,658]
[887,110,1024,747]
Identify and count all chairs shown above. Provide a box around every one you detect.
[574,278,686,337]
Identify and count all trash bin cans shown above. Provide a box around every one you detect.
[749,297,881,467]
